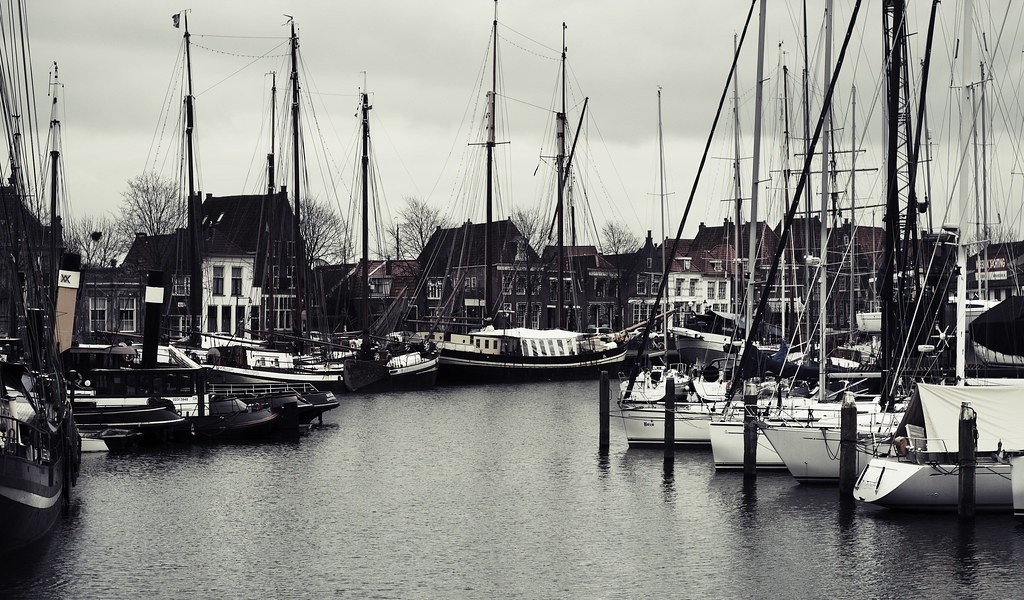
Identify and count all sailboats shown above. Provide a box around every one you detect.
[1,0,1024,565]
